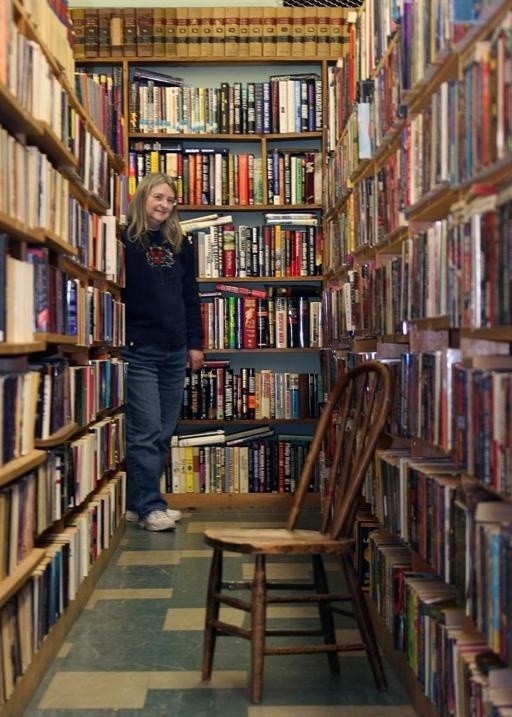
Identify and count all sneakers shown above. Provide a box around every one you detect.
[125,505,182,530]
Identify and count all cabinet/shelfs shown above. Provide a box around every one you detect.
[0,2,509,713]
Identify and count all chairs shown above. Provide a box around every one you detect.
[199,360,396,704]
